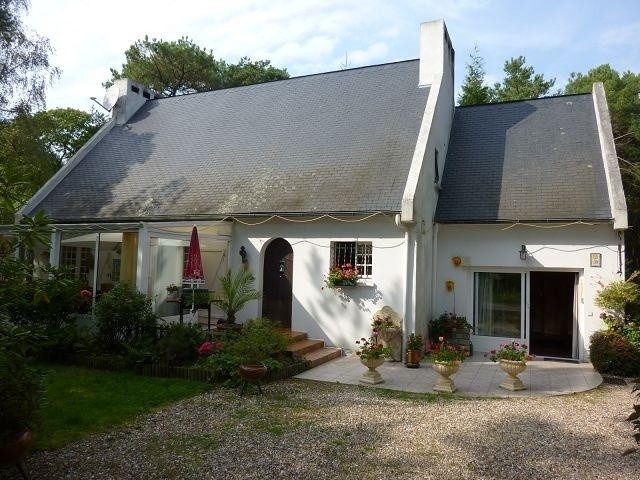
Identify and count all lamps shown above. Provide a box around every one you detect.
[280,258,288,282]
[239,246,247,263]
[518,245,527,260]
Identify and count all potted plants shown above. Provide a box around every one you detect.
[229,342,272,379]
[184,262,267,334]
[166,284,178,297]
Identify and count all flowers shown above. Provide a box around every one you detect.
[428,340,468,366]
[355,334,394,360]
[483,339,536,365]
[405,333,424,350]
[319,263,360,290]
[370,313,403,336]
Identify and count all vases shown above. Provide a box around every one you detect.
[406,349,422,364]
[432,362,461,394]
[498,359,527,391]
[379,327,402,362]
[359,357,385,385]
[333,278,357,286]
